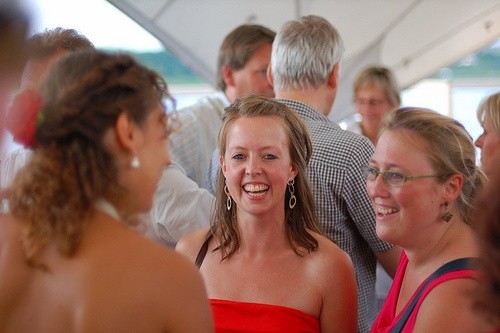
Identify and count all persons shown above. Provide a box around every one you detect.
[472,91,500,183]
[0,51,209,333]
[0,0,30,148]
[0,27,217,249]
[366,108,500,333]
[176,95,358,333]
[207,15,403,333]
[347,67,401,147]
[162,23,277,192]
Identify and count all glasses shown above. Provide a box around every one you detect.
[360,164,453,187]
[353,97,386,108]
